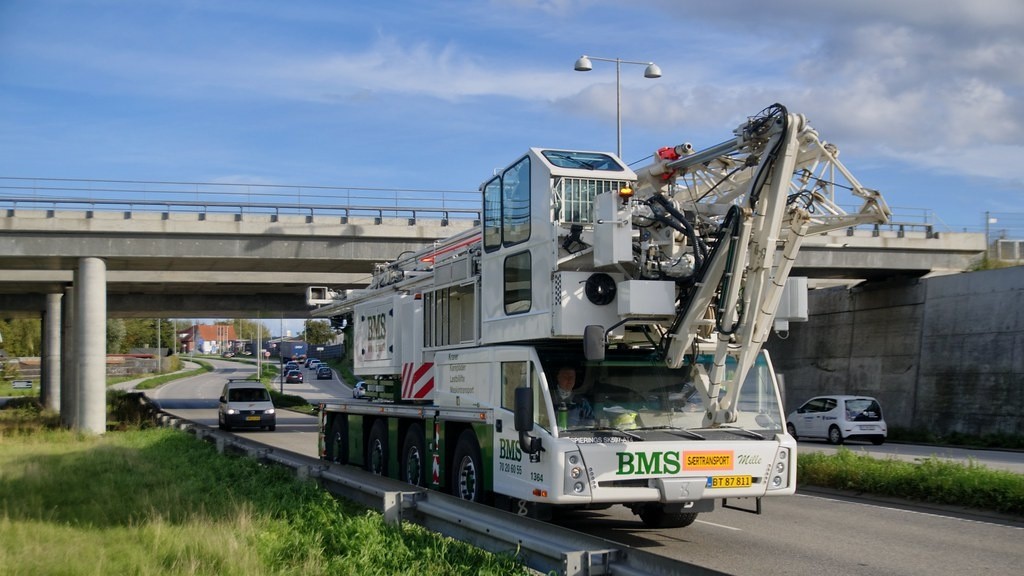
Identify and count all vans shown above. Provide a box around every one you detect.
[219,377,277,432]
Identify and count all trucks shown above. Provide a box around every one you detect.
[278,341,309,364]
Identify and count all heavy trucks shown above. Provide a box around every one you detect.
[306,97,892,534]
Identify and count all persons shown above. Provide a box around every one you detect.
[549,365,597,426]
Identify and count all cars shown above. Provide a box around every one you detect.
[786,395,888,445]
[304,357,327,376]
[223,351,234,358]
[284,361,300,375]
[316,366,332,380]
[352,381,371,398]
[286,371,304,384]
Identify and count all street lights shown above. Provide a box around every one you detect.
[571,55,664,162]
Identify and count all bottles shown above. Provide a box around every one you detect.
[558,402,568,429]
